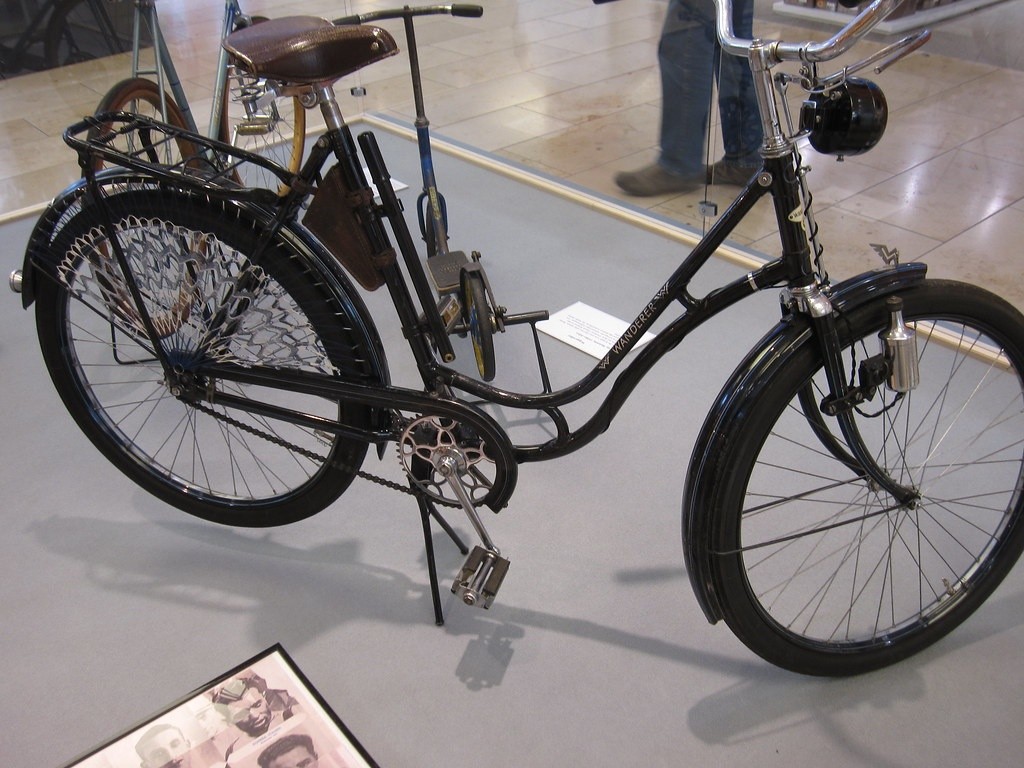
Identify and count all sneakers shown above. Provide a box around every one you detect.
[706,158,761,187]
[613,163,704,195]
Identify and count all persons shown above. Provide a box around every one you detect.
[614,0,765,198]
[256,733,319,768]
[211,675,298,763]
[135,724,226,768]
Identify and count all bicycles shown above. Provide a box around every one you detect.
[329,6,510,381]
[78,0,311,371]
[7,0,1024,679]
